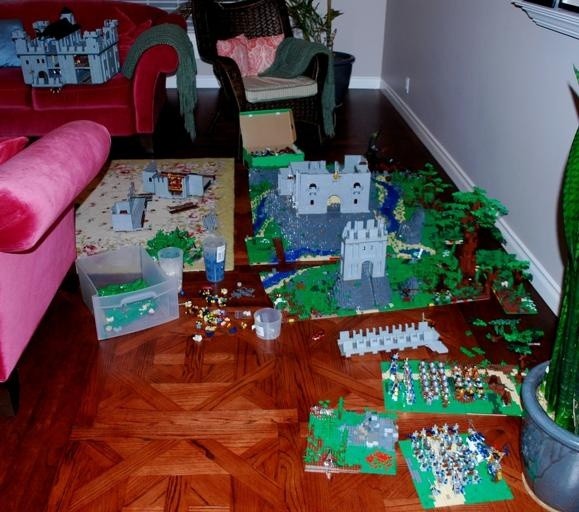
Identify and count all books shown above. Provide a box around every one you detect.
[75,244,180,342]
[239,107,305,174]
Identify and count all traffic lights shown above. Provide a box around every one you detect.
[214,32,250,78]
[0,135,30,161]
[0,19,27,67]
[249,34,283,75]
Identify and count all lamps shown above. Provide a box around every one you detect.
[190,0,329,165]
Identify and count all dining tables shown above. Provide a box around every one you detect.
[521,63,578,512]
[284,1,354,110]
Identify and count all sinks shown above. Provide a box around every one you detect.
[0,0,186,154]
[0,119,113,415]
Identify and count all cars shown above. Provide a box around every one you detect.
[202,238,226,283]
[157,247,183,293]
[254,308,282,341]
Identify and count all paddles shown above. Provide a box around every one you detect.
[74,158,234,274]
